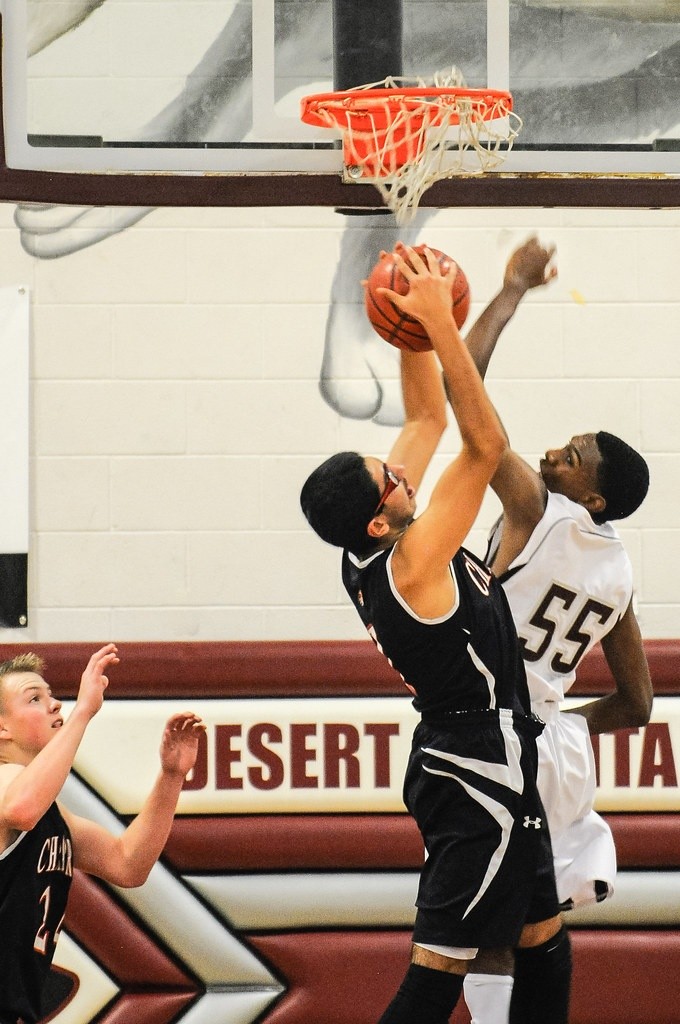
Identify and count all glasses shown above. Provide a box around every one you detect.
[371,462,400,519]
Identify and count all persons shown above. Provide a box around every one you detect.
[443,232,652,1023]
[300,242,571,1024]
[0,642,207,1024]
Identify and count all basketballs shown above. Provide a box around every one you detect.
[365,246,470,353]
